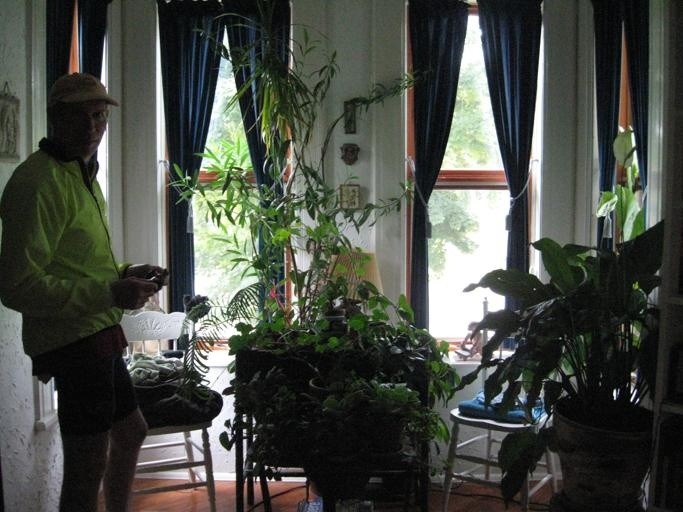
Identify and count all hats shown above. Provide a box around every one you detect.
[48,71,118,110]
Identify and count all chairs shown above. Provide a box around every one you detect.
[443,323,565,512]
[119,310,223,512]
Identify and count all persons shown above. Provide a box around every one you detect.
[0,73,168,512]
[461,323,482,356]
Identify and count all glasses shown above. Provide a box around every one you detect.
[82,107,110,124]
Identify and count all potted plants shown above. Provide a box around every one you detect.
[445,125,664,512]
